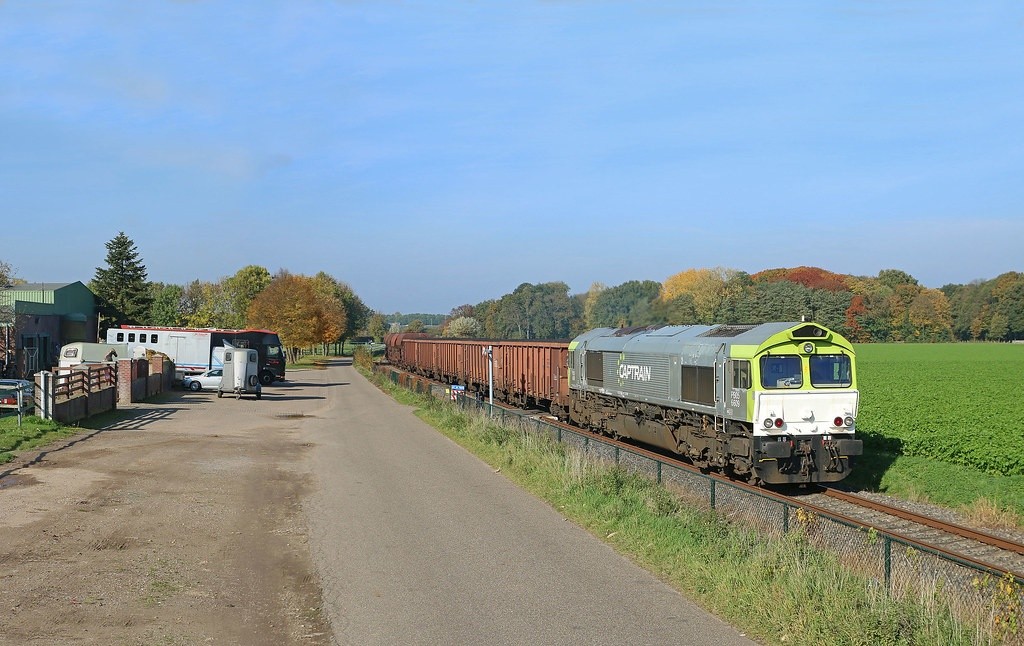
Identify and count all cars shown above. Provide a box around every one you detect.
[183,368,223,391]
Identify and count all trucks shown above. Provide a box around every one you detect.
[107,325,287,386]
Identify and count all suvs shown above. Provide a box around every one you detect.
[0,379,35,415]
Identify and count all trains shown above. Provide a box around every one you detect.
[384,315,864,485]
